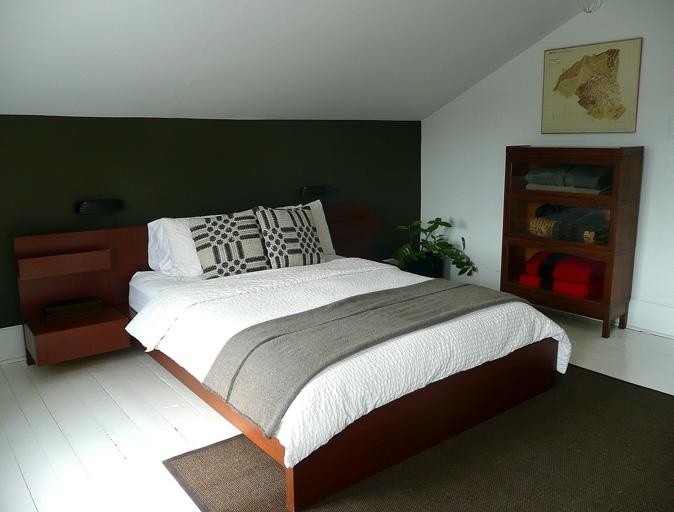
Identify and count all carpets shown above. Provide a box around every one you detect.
[161,361,674,512]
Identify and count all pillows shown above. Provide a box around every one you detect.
[147,218,174,272]
[255,199,336,270]
[161,215,203,278]
[188,208,271,280]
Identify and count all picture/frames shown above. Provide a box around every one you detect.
[541,37,643,134]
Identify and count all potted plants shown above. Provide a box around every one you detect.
[392,217,480,278]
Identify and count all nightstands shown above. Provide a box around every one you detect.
[23,304,129,367]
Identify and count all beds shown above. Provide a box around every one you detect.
[12,204,558,511]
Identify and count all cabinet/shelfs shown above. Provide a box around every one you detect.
[500,144,646,340]
